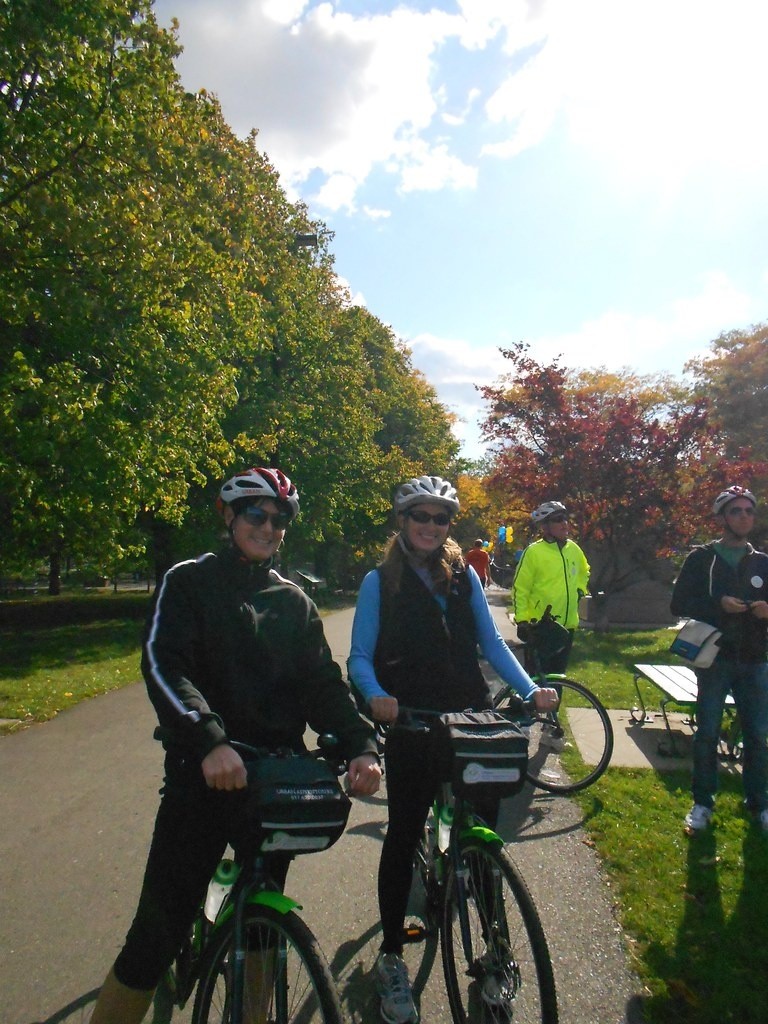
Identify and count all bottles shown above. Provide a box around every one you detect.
[438,804,454,853]
[204,858,238,924]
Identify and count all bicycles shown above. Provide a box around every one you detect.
[493,604,614,793]
[385,699,565,1024]
[175,738,346,1024]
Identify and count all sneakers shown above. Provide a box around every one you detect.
[684,802,712,831]
[756,809,768,831]
[538,730,565,751]
[377,959,419,1024]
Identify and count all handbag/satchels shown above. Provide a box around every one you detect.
[670,618,725,669]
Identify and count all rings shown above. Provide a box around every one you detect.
[549,698,557,702]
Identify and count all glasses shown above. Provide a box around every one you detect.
[244,505,291,529]
[552,514,569,523]
[730,507,756,516]
[408,511,450,525]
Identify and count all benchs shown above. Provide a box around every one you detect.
[628,664,739,757]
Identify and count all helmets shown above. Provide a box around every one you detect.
[536,500,567,521]
[216,468,300,520]
[394,475,460,514]
[713,486,757,513]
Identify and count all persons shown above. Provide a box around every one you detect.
[89,467,383,1024]
[347,474,561,1024]
[670,485,768,830]
[466,538,491,590]
[512,500,592,752]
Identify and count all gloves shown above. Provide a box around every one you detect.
[517,622,531,643]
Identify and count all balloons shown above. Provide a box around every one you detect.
[498,526,513,544]
[482,540,493,552]
